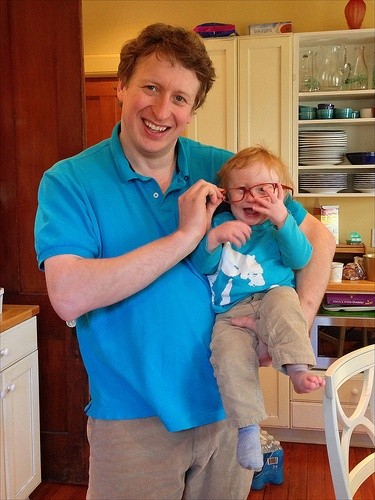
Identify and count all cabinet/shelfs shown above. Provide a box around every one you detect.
[294,29,375,197]
[290,371,370,434]
[0,315,41,500]
[180,33,294,193]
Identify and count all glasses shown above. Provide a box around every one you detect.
[221,182,295,204]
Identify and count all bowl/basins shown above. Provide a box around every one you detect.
[346,152,375,165]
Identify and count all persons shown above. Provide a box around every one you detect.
[191,149,326,473]
[34,24,336,500]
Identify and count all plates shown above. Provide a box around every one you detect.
[299,172,348,193]
[321,298,375,312]
[299,129,348,165]
[353,173,375,193]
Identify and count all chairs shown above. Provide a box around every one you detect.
[324,344,375,500]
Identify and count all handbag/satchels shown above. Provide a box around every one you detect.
[192,22,240,38]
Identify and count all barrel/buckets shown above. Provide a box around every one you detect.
[363,253,375,282]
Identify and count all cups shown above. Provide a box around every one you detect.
[329,262,344,282]
[299,103,375,117]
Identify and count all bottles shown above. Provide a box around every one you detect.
[250,429,283,490]
[300,50,321,91]
[349,46,369,89]
[345,0,366,30]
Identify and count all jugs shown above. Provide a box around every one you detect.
[316,44,351,91]
